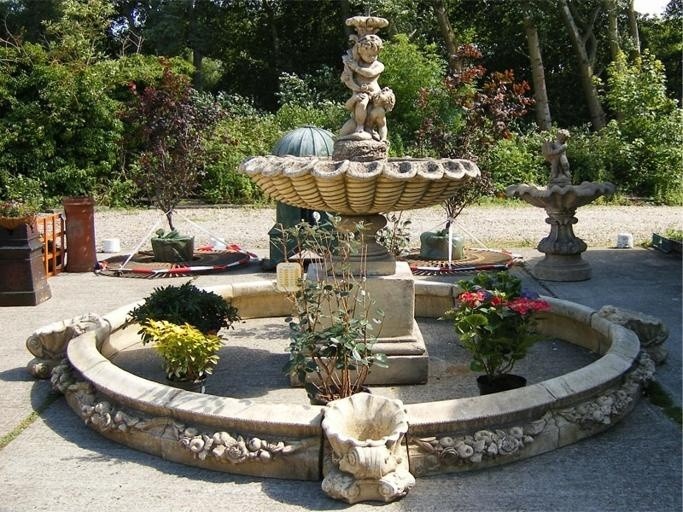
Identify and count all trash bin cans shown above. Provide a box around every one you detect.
[63,199,96,272]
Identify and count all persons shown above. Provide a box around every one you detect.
[546,129,572,178]
[340,35,385,133]
[340,86,396,142]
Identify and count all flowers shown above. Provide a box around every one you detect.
[438,269,549,382]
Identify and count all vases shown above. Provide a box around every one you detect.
[477,375,527,393]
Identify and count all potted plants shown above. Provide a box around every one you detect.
[412,42,536,260]
[122,280,246,392]
[113,57,242,260]
[46,160,112,274]
[269,208,411,405]
[0,168,44,230]
